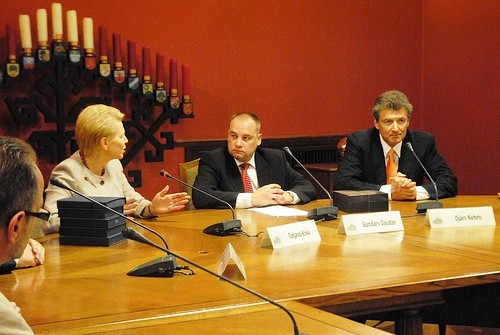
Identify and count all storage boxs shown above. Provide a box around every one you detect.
[60,214,127,227]
[58,223,126,237]
[56,196,126,208]
[57,205,123,220]
[59,233,127,247]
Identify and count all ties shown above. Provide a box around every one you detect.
[386,148,397,184]
[240,163,253,192]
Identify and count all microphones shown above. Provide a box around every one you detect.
[50,177,174,278]
[283,147,338,220]
[160,169,243,236]
[121,227,299,335]
[406,141,443,214]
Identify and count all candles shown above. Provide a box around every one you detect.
[182,63,190,94]
[170,58,177,89]
[156,52,165,82]
[52,3,63,36]
[113,32,122,61]
[99,23,108,55]
[18,14,32,48]
[6,24,16,56]
[142,47,152,75]
[127,39,136,69]
[66,10,78,43]
[36,9,48,42]
[83,16,94,48]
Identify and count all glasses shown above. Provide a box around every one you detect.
[6,208,51,227]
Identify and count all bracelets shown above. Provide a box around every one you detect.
[147,204,154,216]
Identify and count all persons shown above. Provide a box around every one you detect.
[0,238,45,275]
[39,104,192,235]
[192,112,318,211]
[0,136,45,335]
[332,90,459,202]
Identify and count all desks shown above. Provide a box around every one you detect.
[0,192,500,335]
[296,163,339,199]
